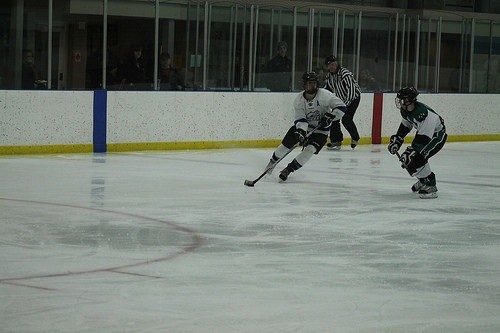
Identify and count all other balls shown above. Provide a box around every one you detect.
[248,184,254,187]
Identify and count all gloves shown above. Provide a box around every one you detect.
[294,128,308,147]
[399,146,417,168]
[318,112,337,128]
[387,134,404,155]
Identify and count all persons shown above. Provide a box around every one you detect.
[323,55,362,150]
[21,49,39,90]
[262,41,294,92]
[388,86,448,199]
[265,71,347,182]
[129,43,183,91]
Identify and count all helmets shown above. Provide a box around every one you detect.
[302,71,319,95]
[395,86,420,109]
[324,55,338,65]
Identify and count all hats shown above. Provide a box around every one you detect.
[278,41,288,50]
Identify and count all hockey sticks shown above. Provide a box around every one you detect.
[394,150,426,178]
[243,123,323,187]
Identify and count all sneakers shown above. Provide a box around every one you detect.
[278,167,291,183]
[327,141,341,150]
[263,159,276,178]
[411,181,426,193]
[419,185,439,199]
[351,140,358,150]
